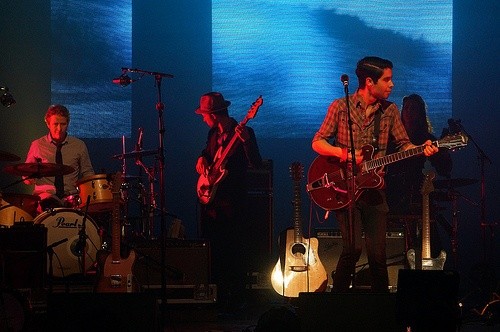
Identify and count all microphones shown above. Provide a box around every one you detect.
[340,74,349,86]
[73,240,84,254]
[113,78,134,84]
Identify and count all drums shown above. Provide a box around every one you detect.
[30,206,104,280]
[73,173,129,212]
[0,192,41,229]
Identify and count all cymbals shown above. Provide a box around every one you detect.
[115,147,159,159]
[0,150,21,162]
[2,162,75,176]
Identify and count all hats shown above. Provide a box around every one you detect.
[195,92,231,114]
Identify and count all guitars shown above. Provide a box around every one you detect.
[93,171,138,293]
[406,170,447,271]
[307,131,470,211]
[196,96,263,204]
[269,161,329,297]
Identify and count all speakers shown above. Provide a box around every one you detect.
[0,227,48,291]
[195,194,273,289]
[297,228,459,332]
[163,239,212,285]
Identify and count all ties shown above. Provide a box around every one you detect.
[51,141,68,200]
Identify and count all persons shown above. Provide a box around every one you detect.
[384,94,452,213]
[312,57,438,293]
[195,92,268,289]
[22,105,94,215]
